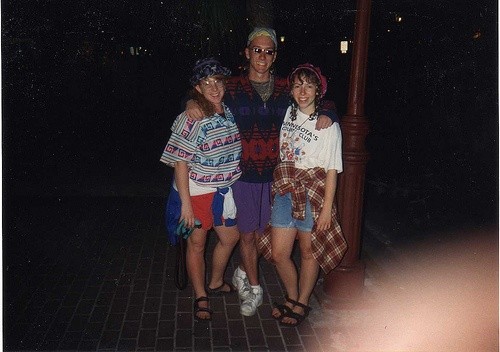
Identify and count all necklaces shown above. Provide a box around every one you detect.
[264,72,272,109]
[283,108,314,147]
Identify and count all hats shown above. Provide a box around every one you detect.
[286,63,327,97]
[189,57,231,88]
[246,26,277,54]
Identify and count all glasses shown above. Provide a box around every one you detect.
[196,78,226,89]
[249,46,276,56]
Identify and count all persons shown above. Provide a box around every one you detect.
[186,28,339,317]
[257,63,348,326]
[160,55,242,321]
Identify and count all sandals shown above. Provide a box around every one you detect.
[271,295,301,319]
[280,301,312,327]
[193,291,211,322]
[209,276,234,295]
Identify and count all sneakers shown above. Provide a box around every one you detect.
[230,266,252,299]
[238,284,264,317]
